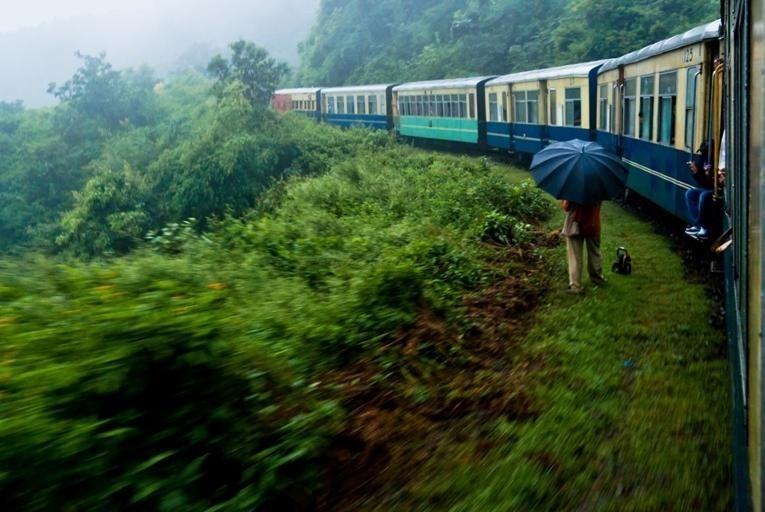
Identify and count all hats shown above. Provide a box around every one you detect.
[696,139,714,153]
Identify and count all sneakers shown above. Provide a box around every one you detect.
[685,226,708,239]
[592,276,604,284]
[571,285,584,292]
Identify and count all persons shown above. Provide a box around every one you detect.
[561,200,608,292]
[683,128,726,240]
[638,112,643,123]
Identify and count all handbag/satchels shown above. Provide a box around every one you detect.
[560,215,579,236]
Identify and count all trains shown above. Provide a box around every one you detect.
[271,0,765,512]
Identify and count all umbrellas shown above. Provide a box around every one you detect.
[530,138,630,201]
